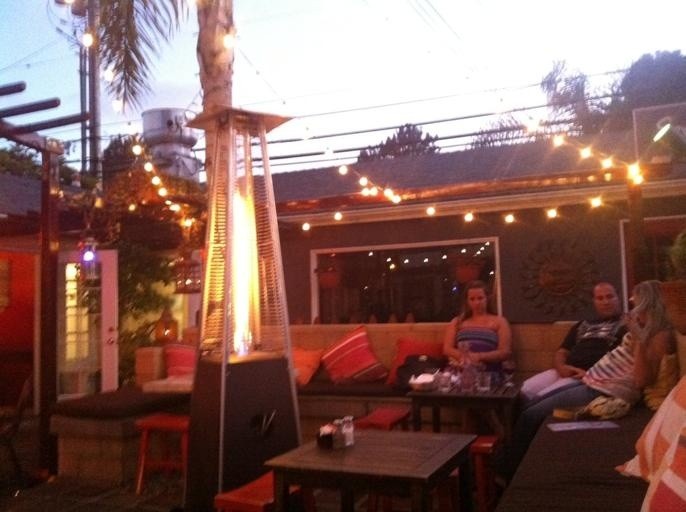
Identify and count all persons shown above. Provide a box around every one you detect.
[512,281,675,455]
[443,279,511,376]
[522,281,629,402]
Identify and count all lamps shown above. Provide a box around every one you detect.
[81,244,95,263]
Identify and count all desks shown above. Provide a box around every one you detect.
[263,432,476,512]
[407,381,519,436]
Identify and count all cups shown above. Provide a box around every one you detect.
[435,375,451,391]
[477,372,491,392]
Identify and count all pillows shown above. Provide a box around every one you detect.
[322,326,386,384]
[384,338,446,387]
[281,344,316,389]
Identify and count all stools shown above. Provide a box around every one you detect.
[132,414,189,494]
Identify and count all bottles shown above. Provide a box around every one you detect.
[332,414,355,451]
[456,348,474,392]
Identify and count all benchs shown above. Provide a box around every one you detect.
[51,380,191,481]
[257,322,578,444]
[466,437,499,512]
[215,403,411,512]
[367,435,498,512]
[498,323,686,512]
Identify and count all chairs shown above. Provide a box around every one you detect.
[0,374,33,490]
